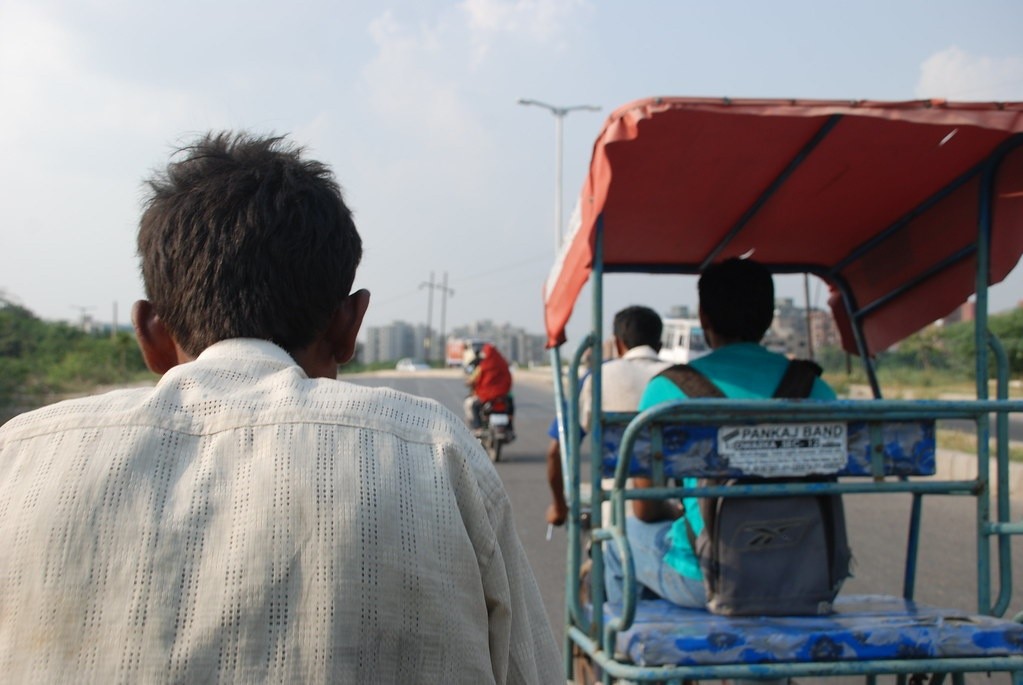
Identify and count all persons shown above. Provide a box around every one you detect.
[466,342,517,443]
[1,130,565,685]
[604,257,849,604]
[546,306,672,596]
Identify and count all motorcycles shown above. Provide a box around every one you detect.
[464,363,516,463]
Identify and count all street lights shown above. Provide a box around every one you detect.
[519,96,602,245]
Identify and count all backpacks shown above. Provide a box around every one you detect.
[647,359,847,615]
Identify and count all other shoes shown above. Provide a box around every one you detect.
[471,428,482,437]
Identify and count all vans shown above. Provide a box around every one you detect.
[660,317,712,367]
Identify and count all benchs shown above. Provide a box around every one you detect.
[577,404,1023,669]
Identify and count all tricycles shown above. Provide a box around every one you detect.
[536,96,1023,685]
